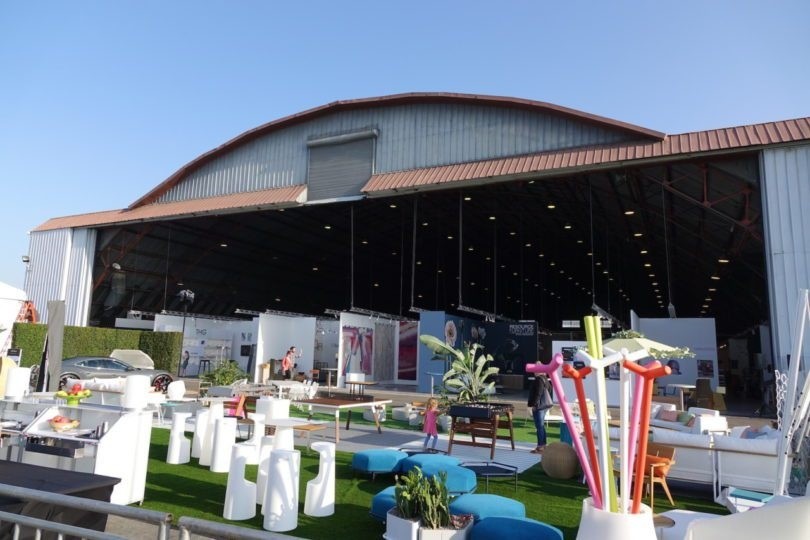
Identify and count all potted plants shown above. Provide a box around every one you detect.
[419,333,516,459]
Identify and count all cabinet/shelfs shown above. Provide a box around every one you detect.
[0,395,155,508]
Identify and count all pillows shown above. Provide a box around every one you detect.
[631,454,670,477]
[678,412,694,424]
[661,409,678,422]
[647,404,660,420]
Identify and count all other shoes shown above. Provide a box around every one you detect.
[530,447,544,454]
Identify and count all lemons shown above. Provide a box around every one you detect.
[57,390,90,397]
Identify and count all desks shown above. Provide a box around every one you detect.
[273,380,300,396]
[322,367,338,398]
[345,379,377,394]
[294,399,392,434]
[230,414,327,449]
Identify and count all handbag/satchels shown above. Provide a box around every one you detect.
[534,375,553,410]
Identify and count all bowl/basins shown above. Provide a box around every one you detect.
[47,418,80,433]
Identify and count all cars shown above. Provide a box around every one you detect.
[58,355,175,396]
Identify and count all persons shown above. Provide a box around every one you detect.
[422,397,448,449]
[282,346,308,383]
[527,360,554,454]
[181,350,189,377]
[16,300,40,324]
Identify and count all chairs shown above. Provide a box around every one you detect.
[612,443,675,509]
[161,380,186,417]
[308,370,321,384]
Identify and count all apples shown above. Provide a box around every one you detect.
[51,415,70,423]
[68,384,81,394]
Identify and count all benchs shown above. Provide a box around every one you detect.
[62,375,163,421]
[582,400,794,498]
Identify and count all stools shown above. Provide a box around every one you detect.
[197,359,210,379]
[354,449,564,540]
[166,397,335,533]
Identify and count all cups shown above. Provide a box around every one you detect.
[574,497,657,540]
